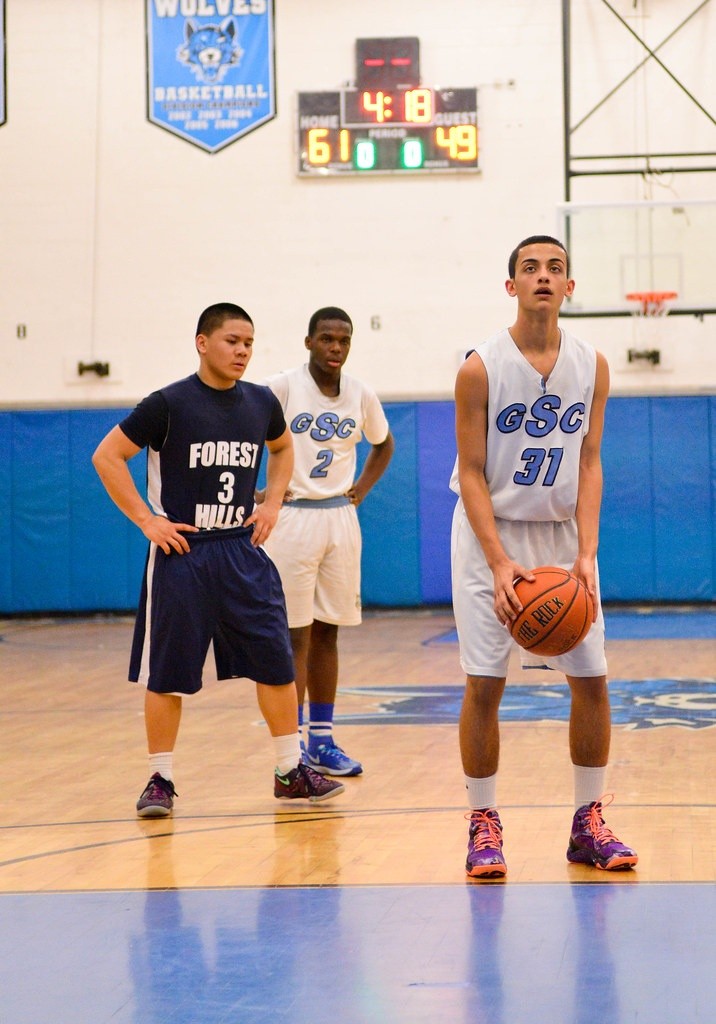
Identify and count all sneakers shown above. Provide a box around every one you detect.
[298,740,364,778]
[273,757,345,802]
[566,792,639,870]
[136,771,179,818]
[463,806,508,879]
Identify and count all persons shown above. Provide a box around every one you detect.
[91,302,346,816]
[449,234,638,879]
[251,307,395,776]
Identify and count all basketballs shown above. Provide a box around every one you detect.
[503,565,595,658]
